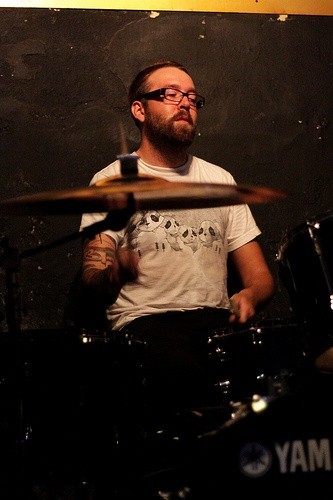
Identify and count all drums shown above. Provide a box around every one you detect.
[274,209,333,321]
[3,328,127,500]
[205,319,308,403]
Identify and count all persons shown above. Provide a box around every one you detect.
[74,58,275,327]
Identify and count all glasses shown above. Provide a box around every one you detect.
[134,88,205,109]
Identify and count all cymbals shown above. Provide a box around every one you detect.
[2,175,279,213]
